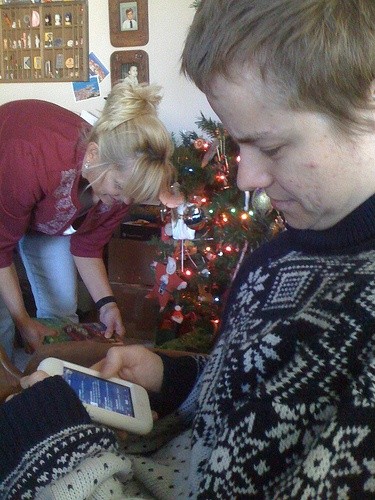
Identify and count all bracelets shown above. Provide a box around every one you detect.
[96,296,116,309]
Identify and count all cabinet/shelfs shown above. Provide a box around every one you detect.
[0,0,89,83]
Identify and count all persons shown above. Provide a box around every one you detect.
[122,8,136,30]
[126,67,139,84]
[0,0,375,500]
[0,78,175,363]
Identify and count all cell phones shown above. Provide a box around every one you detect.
[38,357,154,434]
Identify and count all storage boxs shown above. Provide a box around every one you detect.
[108,202,165,285]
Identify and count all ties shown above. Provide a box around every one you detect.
[129,21,133,28]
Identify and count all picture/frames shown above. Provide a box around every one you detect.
[110,49,149,91]
[108,0,149,45]
